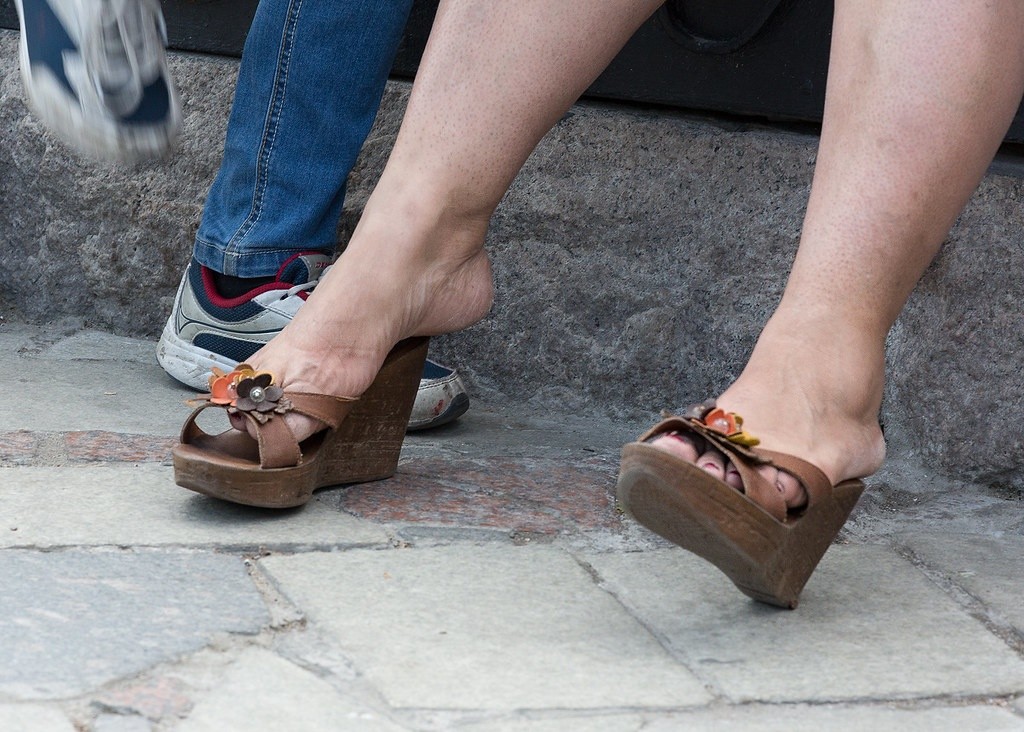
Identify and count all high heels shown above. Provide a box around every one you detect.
[615,399,866,609]
[172,334,432,507]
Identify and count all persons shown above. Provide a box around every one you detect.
[175,0,1024,608]
[14,0,470,433]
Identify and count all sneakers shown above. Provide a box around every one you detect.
[12,0,180,161]
[153,249,468,431]
